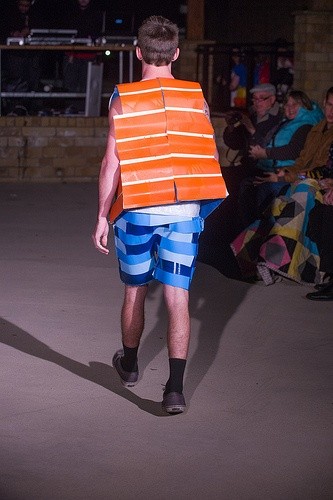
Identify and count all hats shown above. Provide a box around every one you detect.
[249,83,276,95]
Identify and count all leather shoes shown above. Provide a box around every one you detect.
[306,282,333,300]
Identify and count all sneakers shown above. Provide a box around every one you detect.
[112,348,138,387]
[161,383,186,413]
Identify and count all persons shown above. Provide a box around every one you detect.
[92,15,227,412]
[0,0,108,117]
[218,43,333,300]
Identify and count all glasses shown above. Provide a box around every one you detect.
[252,94,272,102]
